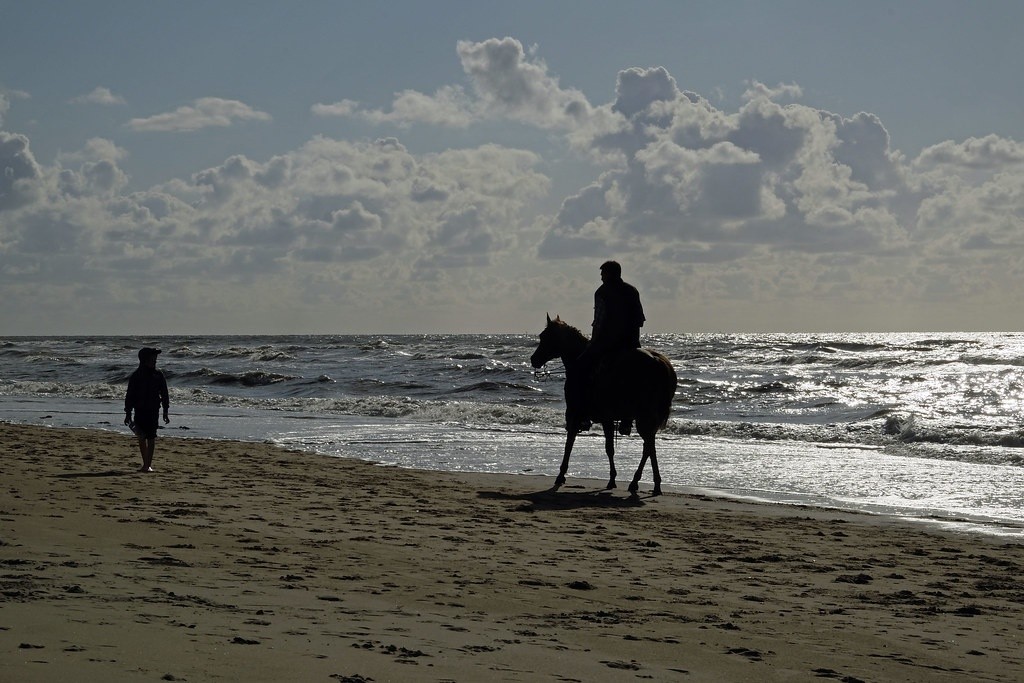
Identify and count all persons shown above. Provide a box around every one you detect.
[123,347,170,472]
[591,260,645,435]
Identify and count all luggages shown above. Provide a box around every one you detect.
[580,420,591,431]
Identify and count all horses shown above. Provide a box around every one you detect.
[531,312,677,494]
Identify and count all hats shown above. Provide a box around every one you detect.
[138,348,162,357]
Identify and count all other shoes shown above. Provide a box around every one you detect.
[619,419,631,435]
